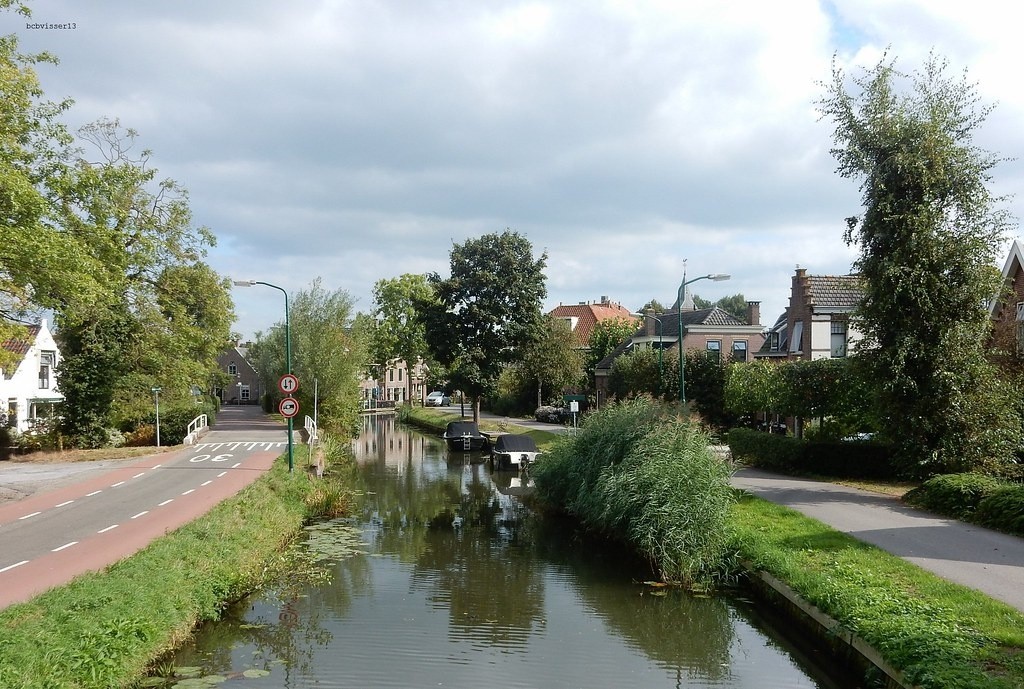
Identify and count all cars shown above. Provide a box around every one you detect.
[424,390,452,407]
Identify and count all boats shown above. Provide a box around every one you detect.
[442,420,487,452]
[490,433,542,471]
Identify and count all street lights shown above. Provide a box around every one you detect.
[629,312,663,399]
[233,280,295,475]
[677,272,732,406]
